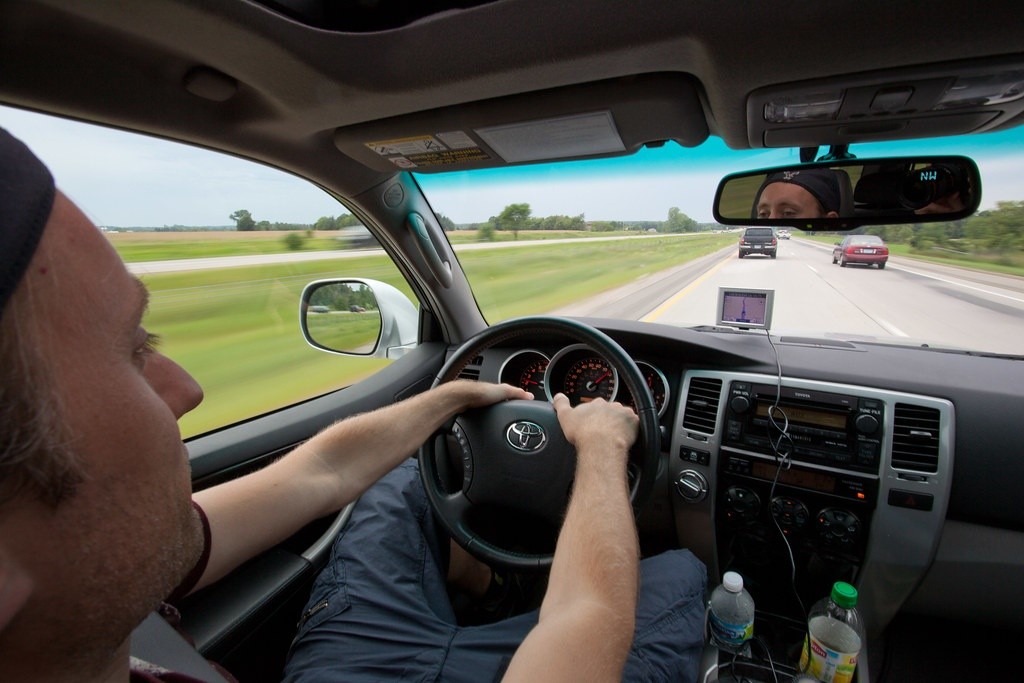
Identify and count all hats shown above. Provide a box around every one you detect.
[751,168,841,218]
[0,125,55,309]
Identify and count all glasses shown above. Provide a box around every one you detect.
[898,167,956,209]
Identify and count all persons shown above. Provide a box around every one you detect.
[755,165,967,219]
[0,126,710,683]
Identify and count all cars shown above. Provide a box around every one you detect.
[832,235,889,269]
[775,229,791,240]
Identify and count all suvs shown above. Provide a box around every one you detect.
[738,226,778,259]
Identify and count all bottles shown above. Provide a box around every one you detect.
[797,582,862,683]
[709,572,754,652]
